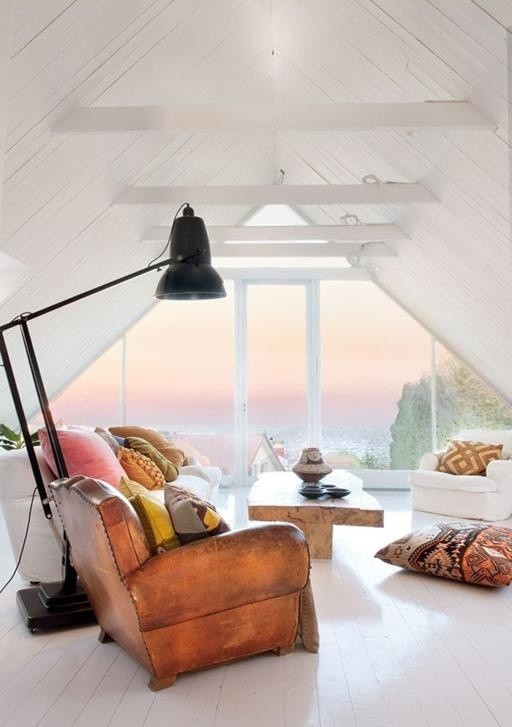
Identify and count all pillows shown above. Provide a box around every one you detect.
[374,519,511,588]
[37,427,231,556]
[438,438,503,477]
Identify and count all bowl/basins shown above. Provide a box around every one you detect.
[298,484,351,498]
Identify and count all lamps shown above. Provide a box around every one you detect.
[0,199,226,638]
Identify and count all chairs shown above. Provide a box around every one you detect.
[409,428,511,521]
[47,474,311,692]
